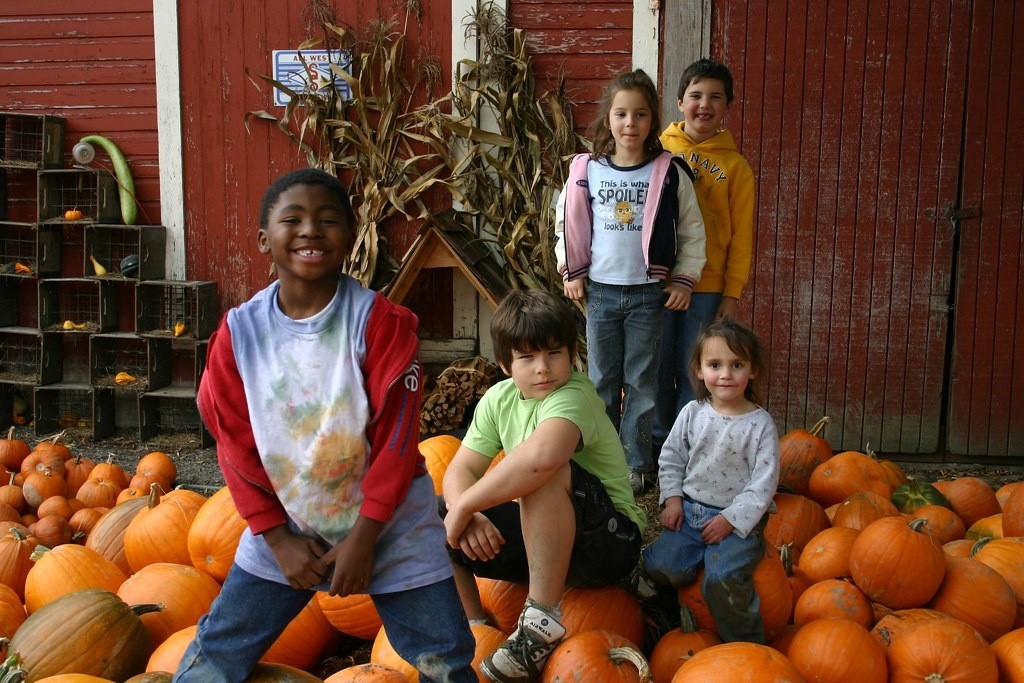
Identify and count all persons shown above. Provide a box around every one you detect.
[555,68,706,495]
[652,59,755,470]
[642,317,780,643]
[435,288,647,683]
[173,168,479,683]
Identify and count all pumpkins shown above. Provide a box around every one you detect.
[0,204,188,426]
[0,416,1024,683]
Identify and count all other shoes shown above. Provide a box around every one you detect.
[630,472,657,495]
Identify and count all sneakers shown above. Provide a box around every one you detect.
[478,595,566,683]
[469,617,492,626]
